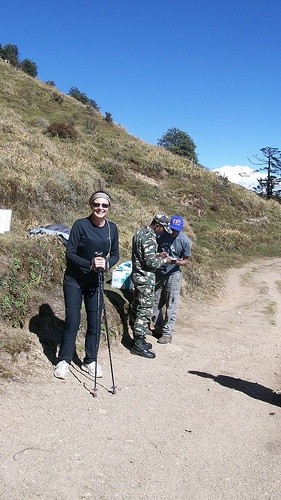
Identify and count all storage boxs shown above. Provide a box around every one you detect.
[111,270,132,288]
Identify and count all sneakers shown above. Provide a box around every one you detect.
[130,343,156,358]
[81,362,103,378]
[132,340,152,349]
[54,360,69,380]
[157,335,172,343]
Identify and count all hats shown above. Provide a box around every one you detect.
[89,191,111,204]
[169,215,183,231]
[153,215,172,234]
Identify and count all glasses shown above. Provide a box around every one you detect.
[91,203,109,208]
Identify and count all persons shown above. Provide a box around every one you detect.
[131,214,173,358]
[54,191,119,379]
[152,216,191,343]
